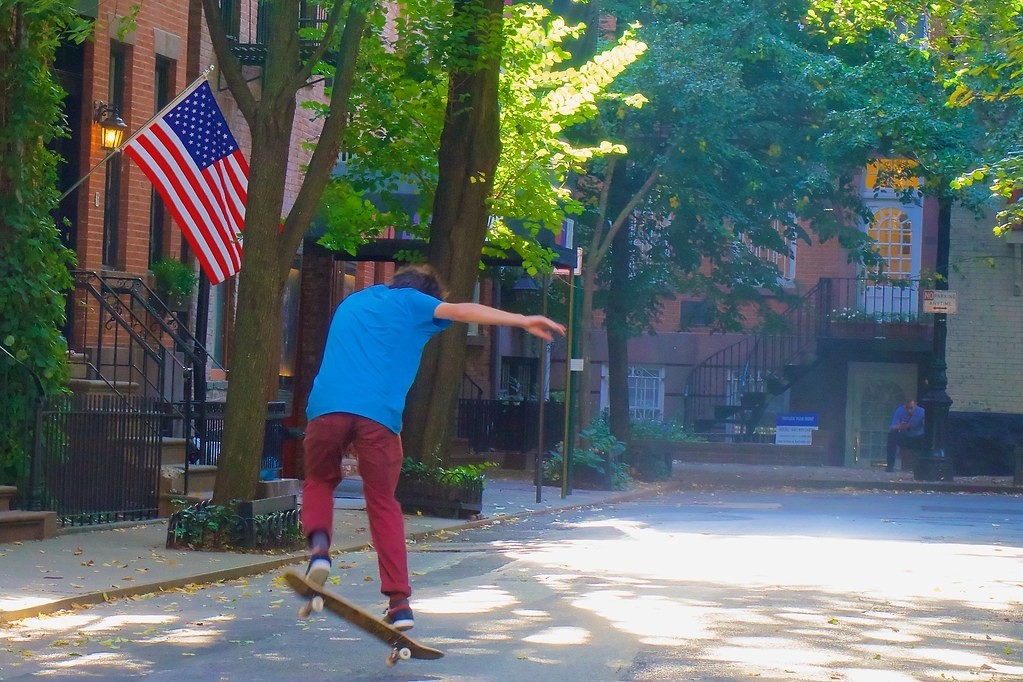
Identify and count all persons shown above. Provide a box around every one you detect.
[885,397,925,472]
[302,266,567,631]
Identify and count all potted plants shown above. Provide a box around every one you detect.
[149,256,201,312]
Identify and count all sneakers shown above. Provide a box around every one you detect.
[380,605,414,632]
[305,548,332,587]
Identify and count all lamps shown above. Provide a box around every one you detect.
[93,99,128,150]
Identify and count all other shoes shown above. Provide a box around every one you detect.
[886,464,893,472]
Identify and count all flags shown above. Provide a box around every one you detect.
[122,78,284,285]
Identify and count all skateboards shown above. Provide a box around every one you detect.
[284,569,444,665]
